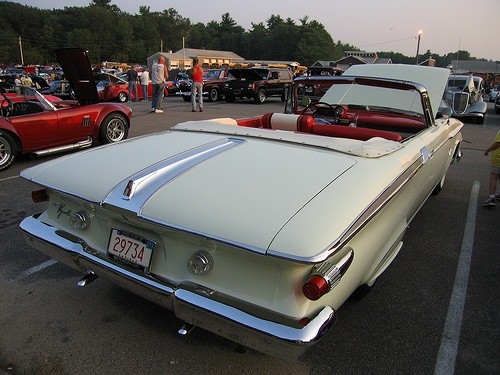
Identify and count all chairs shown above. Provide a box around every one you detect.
[262,113,402,143]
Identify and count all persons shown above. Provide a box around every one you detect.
[127,65,139,103]
[188,58,204,112]
[139,67,149,103]
[14,72,35,88]
[292,66,299,73]
[151,56,169,113]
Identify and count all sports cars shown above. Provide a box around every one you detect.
[0,47,134,173]
[15,63,464,363]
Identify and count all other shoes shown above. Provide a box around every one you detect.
[495,193,500,200]
[155,109,163,113]
[481,197,495,207]
[192,108,196,112]
[199,108,204,111]
[152,107,155,111]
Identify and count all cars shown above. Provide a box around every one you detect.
[438,74,487,124]
[488,84,499,103]
[1,59,346,104]
[493,91,500,114]
